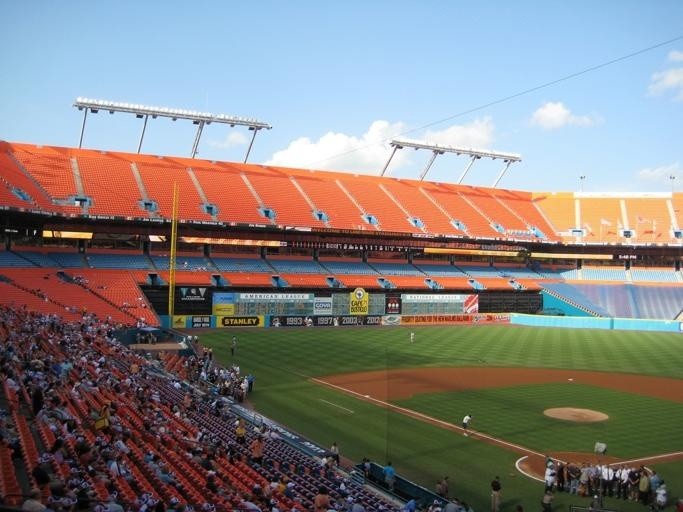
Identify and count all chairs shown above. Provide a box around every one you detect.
[0,352,398,512]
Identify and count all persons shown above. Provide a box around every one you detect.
[463,414,472,437]
[183,263,208,272]
[1,300,373,512]
[382,461,474,512]
[491,476,500,512]
[33,271,106,303]
[541,456,667,512]
[410,332,415,343]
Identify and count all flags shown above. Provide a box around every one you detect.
[638,216,647,224]
[602,219,610,227]
